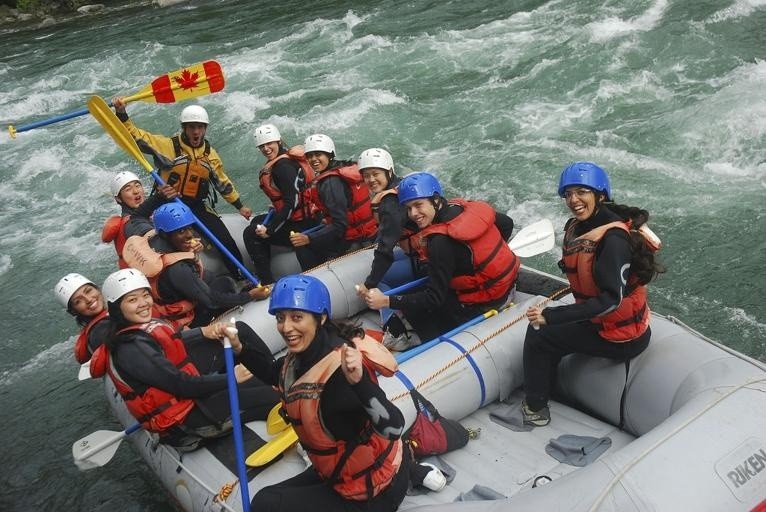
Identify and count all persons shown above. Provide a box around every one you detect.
[102,172,154,270]
[490,163,667,431]
[218,275,447,512]
[354,147,430,326]
[289,135,378,272]
[55,273,189,388]
[90,268,282,453]
[111,95,252,288]
[121,185,272,329]
[243,123,322,286]
[365,172,520,351]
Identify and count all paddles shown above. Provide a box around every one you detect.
[377,220,554,295]
[8,60,224,139]
[87,96,271,295]
[246,302,513,466]
[72,421,141,470]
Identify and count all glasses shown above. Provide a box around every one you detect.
[563,188,593,198]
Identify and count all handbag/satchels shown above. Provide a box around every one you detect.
[404,411,469,457]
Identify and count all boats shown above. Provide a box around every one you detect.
[96,209,766,512]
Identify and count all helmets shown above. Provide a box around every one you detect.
[53,268,152,312]
[358,147,395,174]
[254,124,280,147]
[303,134,336,157]
[180,104,210,124]
[111,171,141,198]
[152,202,196,233]
[558,162,611,199]
[398,172,441,205]
[268,274,332,320]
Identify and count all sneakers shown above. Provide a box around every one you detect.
[521,401,550,426]
[419,462,446,492]
[382,330,410,352]
